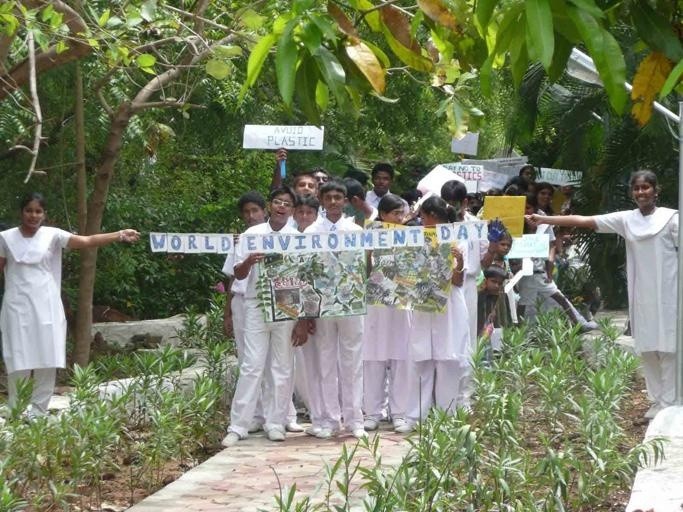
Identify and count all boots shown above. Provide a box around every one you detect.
[573,318,598,335]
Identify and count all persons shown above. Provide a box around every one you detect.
[440,180,507,360]
[516,208,598,334]
[301,182,373,440]
[307,168,331,189]
[342,179,378,230]
[394,196,470,433]
[269,148,319,205]
[293,193,325,436]
[524,170,680,419]
[364,196,411,433]
[476,265,513,338]
[0,192,141,420]
[487,230,517,324]
[366,163,409,218]
[534,181,563,257]
[466,165,538,217]
[557,226,602,321]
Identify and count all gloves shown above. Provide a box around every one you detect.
[488,217,506,241]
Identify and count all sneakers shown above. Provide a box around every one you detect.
[394,418,403,426]
[268,428,286,441]
[644,404,661,419]
[316,427,336,439]
[353,428,370,440]
[308,426,323,435]
[365,420,378,431]
[248,420,262,433]
[222,433,241,448]
[396,426,415,434]
[287,421,306,433]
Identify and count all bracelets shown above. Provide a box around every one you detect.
[118,229,123,242]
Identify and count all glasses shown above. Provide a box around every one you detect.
[273,198,290,208]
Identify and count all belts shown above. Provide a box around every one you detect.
[534,270,547,273]
